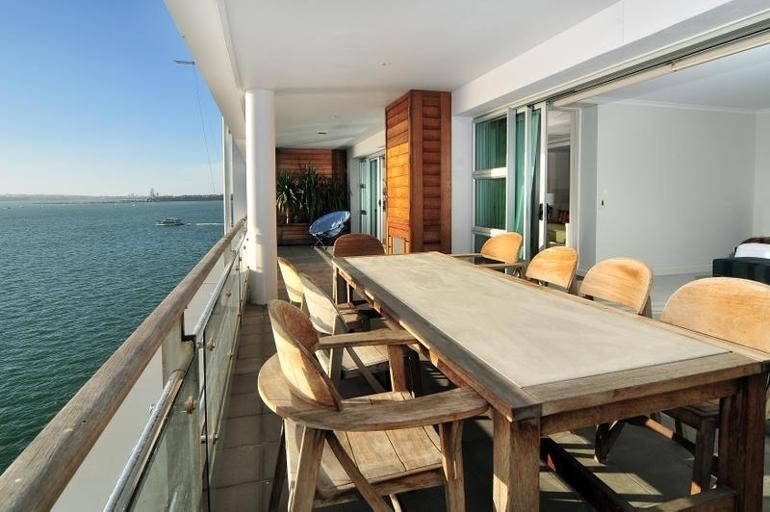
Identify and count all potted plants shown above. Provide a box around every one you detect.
[275,160,344,224]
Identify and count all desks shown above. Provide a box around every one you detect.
[326,250,770,511]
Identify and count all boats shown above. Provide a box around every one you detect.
[154,217,185,227]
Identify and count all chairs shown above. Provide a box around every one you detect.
[297,271,422,395]
[449,232,528,275]
[332,233,385,305]
[309,210,351,250]
[519,245,578,296]
[594,275,770,491]
[276,256,371,330]
[255,298,490,511]
[579,257,653,319]
